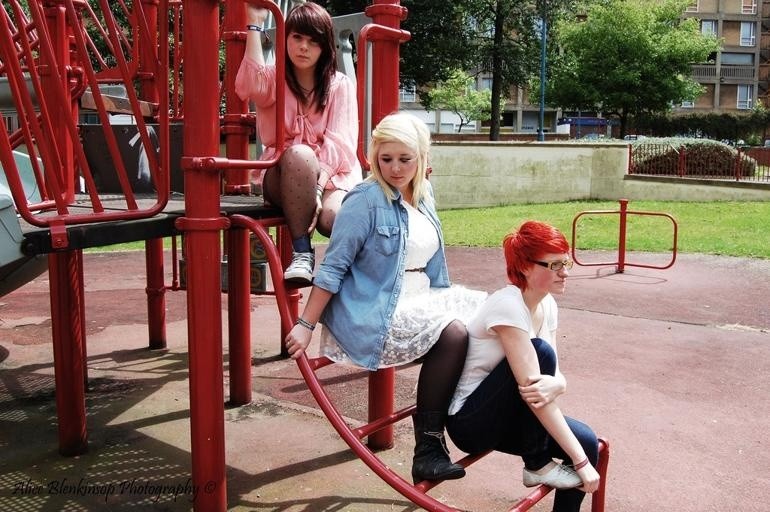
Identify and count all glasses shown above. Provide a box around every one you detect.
[533,257,577,272]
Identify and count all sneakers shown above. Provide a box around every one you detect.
[284,246,315,286]
[522,464,584,490]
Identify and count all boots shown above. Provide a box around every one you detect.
[410,409,466,484]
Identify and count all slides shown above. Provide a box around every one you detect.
[0,150,48,297]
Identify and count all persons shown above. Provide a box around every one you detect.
[235,0,363,288]
[445,220,602,512]
[282,114,490,486]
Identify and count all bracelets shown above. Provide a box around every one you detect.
[294,317,315,333]
[247,24,262,32]
[316,183,324,200]
[571,456,589,472]
